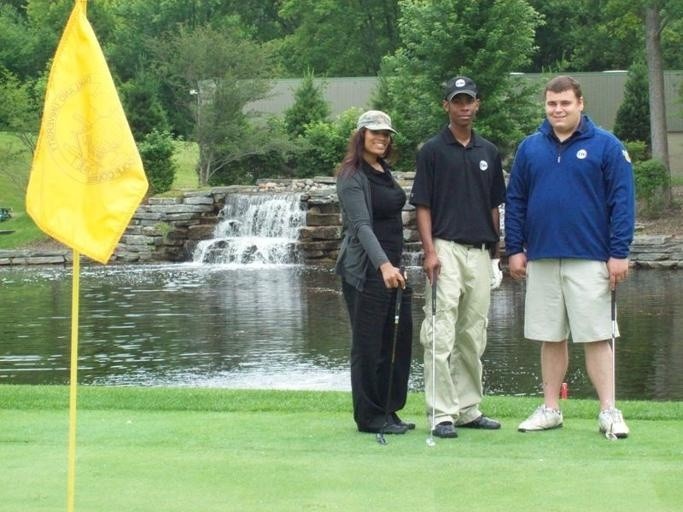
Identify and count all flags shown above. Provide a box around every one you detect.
[24,0,152,266]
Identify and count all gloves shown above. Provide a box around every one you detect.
[490,258,504,290]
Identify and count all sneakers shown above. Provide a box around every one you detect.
[518,405,563,432]
[599,408,629,440]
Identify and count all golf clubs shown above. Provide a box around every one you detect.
[606,290,618,441]
[377,264,406,443]
[425,265,437,447]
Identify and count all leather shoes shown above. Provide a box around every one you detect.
[462,416,500,429]
[379,421,416,434]
[432,422,457,438]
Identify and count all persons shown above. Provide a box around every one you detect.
[336,110,416,433]
[505,76,634,437]
[409,76,505,436]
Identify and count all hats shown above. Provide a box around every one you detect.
[445,76,477,102]
[357,110,398,135]
